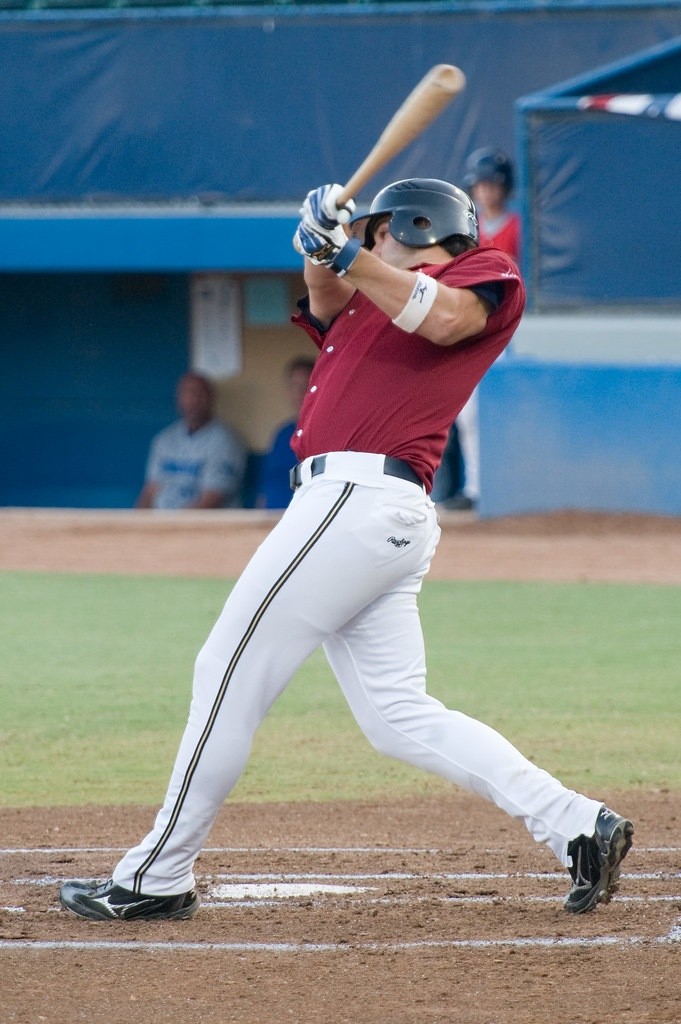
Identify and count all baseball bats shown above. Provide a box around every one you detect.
[292,61,466,252]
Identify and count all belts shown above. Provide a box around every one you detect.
[289,454,424,490]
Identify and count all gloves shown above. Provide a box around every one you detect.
[303,182,355,233]
[292,214,362,278]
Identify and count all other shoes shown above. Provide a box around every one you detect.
[440,491,473,508]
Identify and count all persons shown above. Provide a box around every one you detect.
[433,145,522,511]
[141,356,318,510]
[59,180,635,923]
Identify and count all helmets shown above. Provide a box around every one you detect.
[349,174,479,249]
[462,147,514,190]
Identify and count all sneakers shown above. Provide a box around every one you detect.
[59,874,200,919]
[562,806,634,914]
